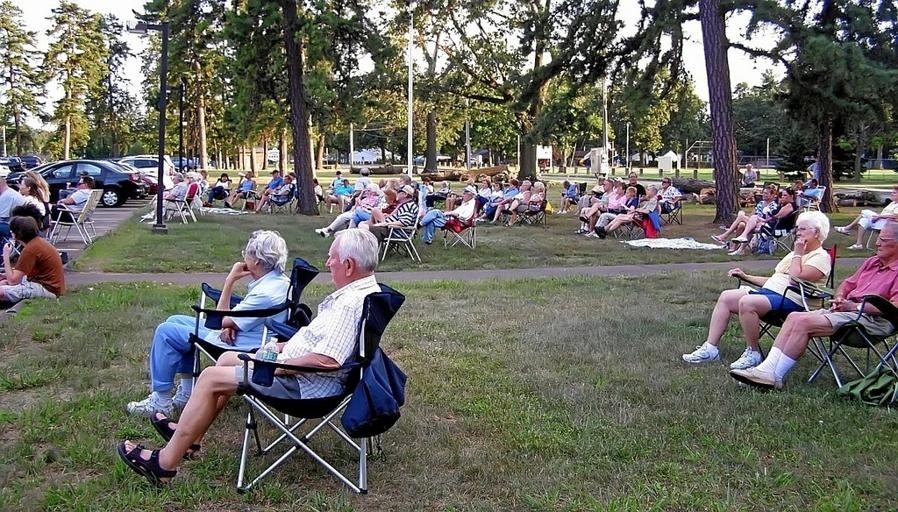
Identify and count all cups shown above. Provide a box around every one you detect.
[67,182,71,189]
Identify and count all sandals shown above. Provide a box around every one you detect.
[580,216,590,223]
[117,440,177,488]
[149,413,199,460]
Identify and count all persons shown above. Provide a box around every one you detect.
[733,218,897,391]
[693,165,819,257]
[833,185,897,250]
[683,210,831,368]
[0,164,66,304]
[313,168,546,256]
[126,229,297,416]
[557,172,682,240]
[116,229,382,490]
[806,157,821,185]
[58,170,90,201]
[47,175,94,234]
[141,169,298,225]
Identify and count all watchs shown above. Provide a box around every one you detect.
[856,301,866,316]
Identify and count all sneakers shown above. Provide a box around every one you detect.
[731,237,748,243]
[202,202,213,207]
[729,366,782,389]
[683,343,719,362]
[728,250,743,256]
[315,229,330,238]
[835,226,849,235]
[710,235,726,246]
[574,227,606,239]
[847,244,863,250]
[127,395,174,415]
[730,349,761,369]
[224,201,232,207]
[172,386,190,406]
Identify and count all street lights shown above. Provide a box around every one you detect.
[122,18,170,233]
[163,81,183,175]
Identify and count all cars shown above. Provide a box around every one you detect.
[0,154,42,178]
[6,160,143,208]
[117,154,198,198]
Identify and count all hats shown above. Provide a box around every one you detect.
[395,185,415,195]
[463,186,478,196]
[270,170,278,174]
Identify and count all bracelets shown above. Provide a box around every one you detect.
[791,255,801,259]
[280,358,288,374]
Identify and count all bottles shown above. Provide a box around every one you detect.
[264,337,281,363]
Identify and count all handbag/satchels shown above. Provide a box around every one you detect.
[342,349,408,438]
[757,239,774,254]
